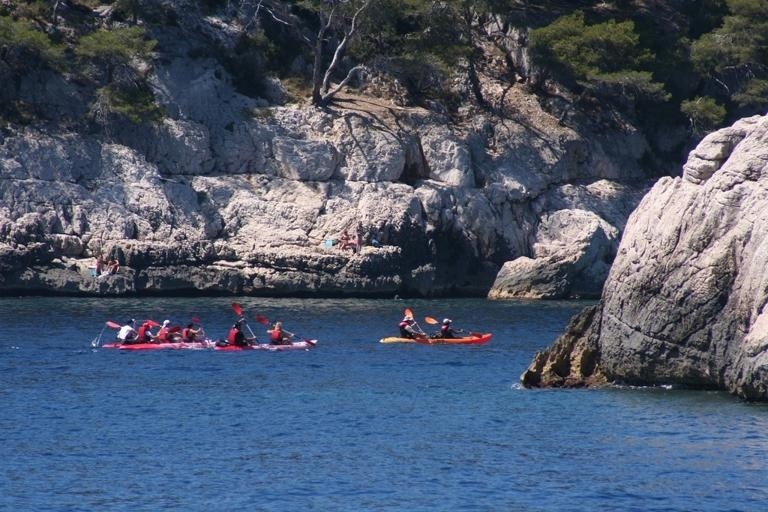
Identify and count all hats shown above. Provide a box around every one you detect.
[126,319,240,328]
[402,316,452,324]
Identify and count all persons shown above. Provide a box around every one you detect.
[157,320,182,344]
[94,257,104,274]
[227,318,256,347]
[105,256,119,275]
[265,322,294,345]
[116,319,138,345]
[439,318,463,339]
[398,315,425,339]
[181,323,203,343]
[355,219,364,256]
[136,322,159,343]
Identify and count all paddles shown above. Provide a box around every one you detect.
[405,308,432,343]
[149,326,180,342]
[231,303,260,345]
[145,320,183,335]
[257,315,316,346]
[425,317,483,338]
[106,321,123,329]
[193,316,216,353]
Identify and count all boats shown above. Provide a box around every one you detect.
[211,336,321,352]
[374,329,496,346]
[100,336,260,352]
